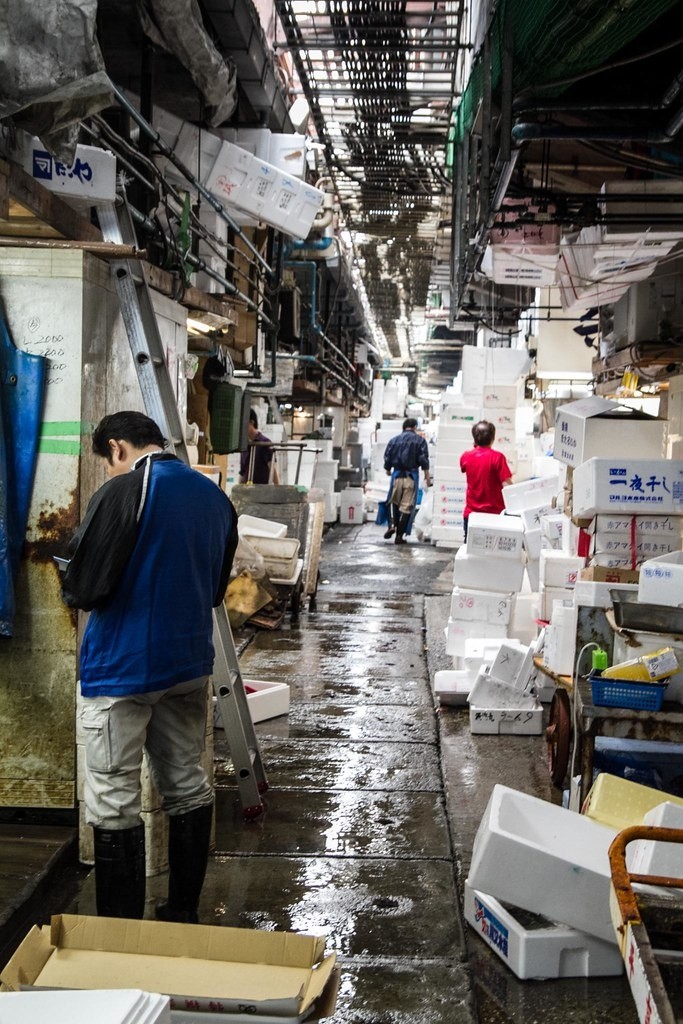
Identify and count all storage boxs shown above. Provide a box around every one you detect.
[192,180,683,979]
[0,914,342,1024]
[0,84,324,346]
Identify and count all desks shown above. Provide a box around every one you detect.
[533,653,683,805]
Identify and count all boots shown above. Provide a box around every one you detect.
[395,510,411,544]
[384,503,398,538]
[94,820,146,919]
[156,802,213,923]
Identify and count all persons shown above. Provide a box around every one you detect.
[384,418,433,544]
[60,411,239,924]
[238,408,280,485]
[460,420,513,544]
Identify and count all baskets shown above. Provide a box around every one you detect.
[586,671,670,712]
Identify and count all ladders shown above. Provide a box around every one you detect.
[97,173,271,816]
